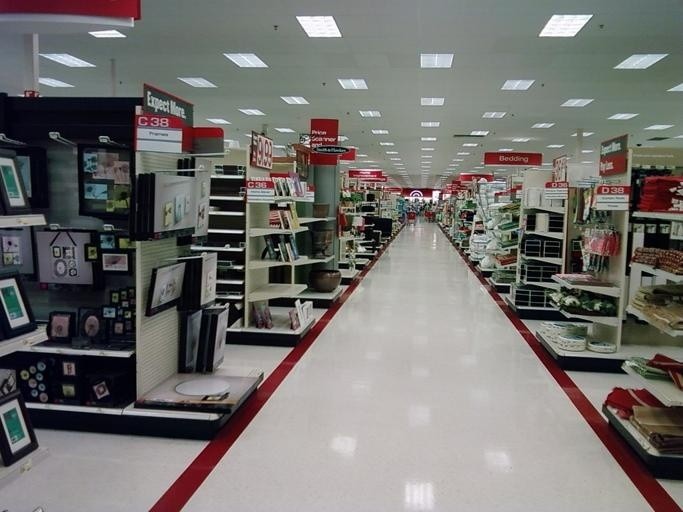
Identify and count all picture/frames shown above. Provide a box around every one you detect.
[1,388,39,466]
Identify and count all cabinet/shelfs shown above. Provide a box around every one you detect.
[194,125,343,342]
[1,85,265,425]
[537,149,632,365]
[340,168,402,284]
[437,147,598,315]
[602,149,680,477]
